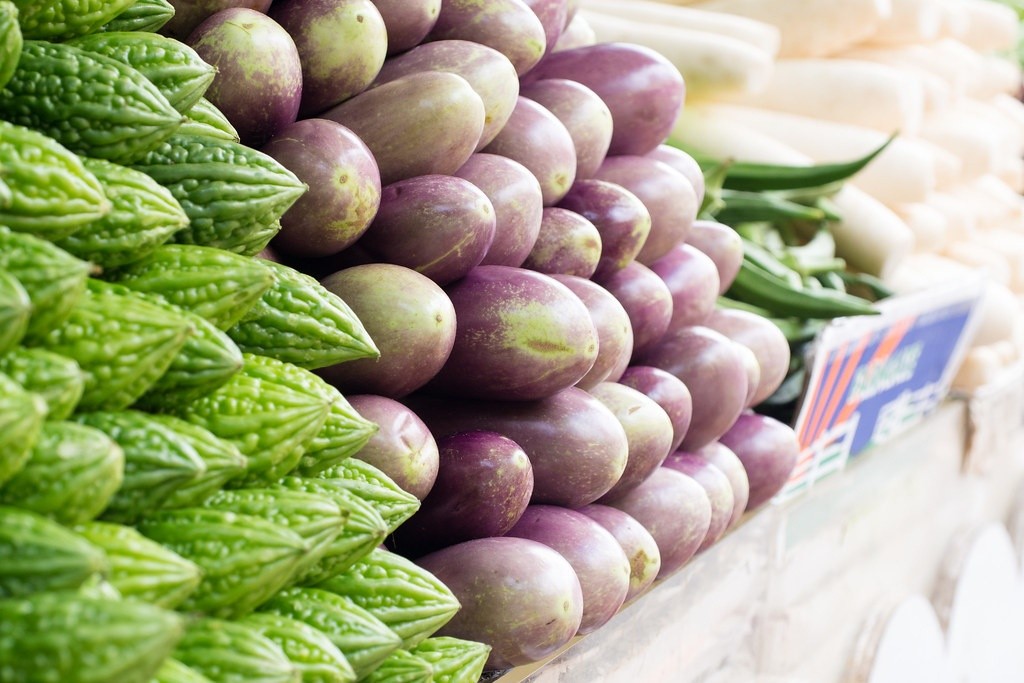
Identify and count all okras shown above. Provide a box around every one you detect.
[664,123,903,375]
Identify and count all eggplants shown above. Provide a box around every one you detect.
[170,0,801,671]
